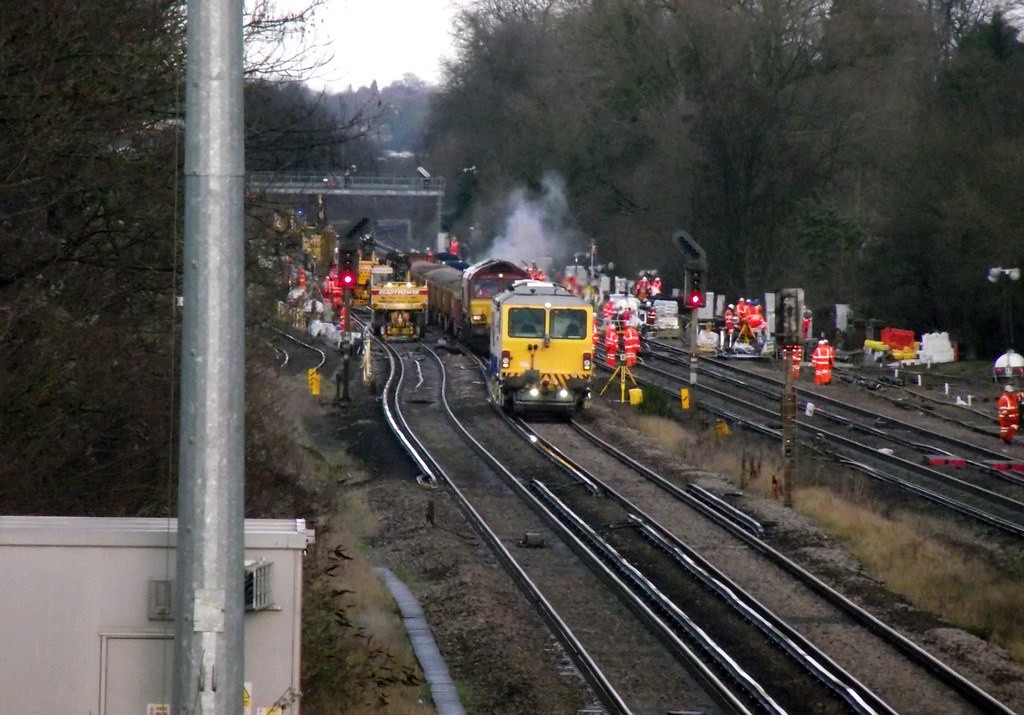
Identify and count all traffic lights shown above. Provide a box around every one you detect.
[685,264,707,307]
[336,248,359,288]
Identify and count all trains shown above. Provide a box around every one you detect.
[402,249,532,346]
[485,279,593,419]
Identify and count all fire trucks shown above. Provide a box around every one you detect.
[299,225,431,342]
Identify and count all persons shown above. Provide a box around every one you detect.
[523,261,766,368]
[450,237,459,255]
[781,311,835,385]
[299,265,345,332]
[998,385,1024,445]
[345,166,357,190]
[477,284,493,297]
[425,246,434,262]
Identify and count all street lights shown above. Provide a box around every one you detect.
[987,267,1021,349]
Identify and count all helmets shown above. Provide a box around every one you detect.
[739,298,745,302]
[625,322,630,326]
[625,305,630,308]
[610,298,614,302]
[567,273,573,278]
[646,302,651,307]
[610,324,616,329]
[529,268,533,270]
[1005,385,1014,392]
[643,277,647,282]
[655,277,660,281]
[755,299,759,304]
[426,247,430,250]
[746,299,751,302]
[818,340,825,344]
[752,302,758,306]
[728,304,735,311]
[593,312,597,317]
[823,339,829,343]
[538,268,543,272]
